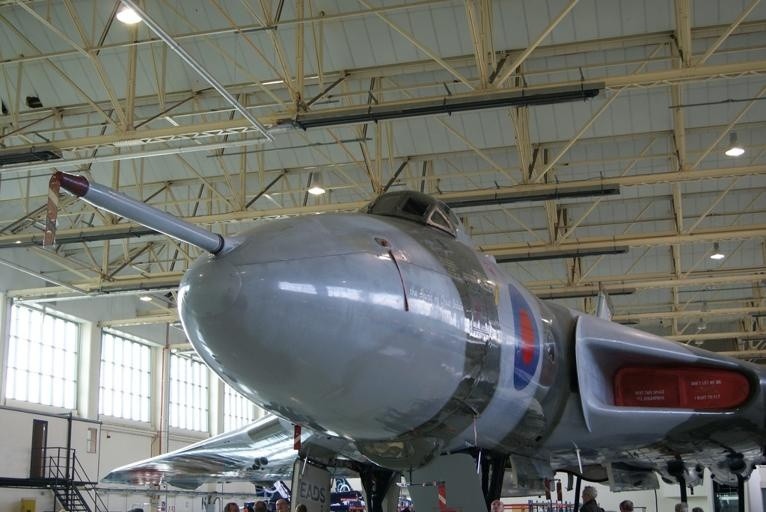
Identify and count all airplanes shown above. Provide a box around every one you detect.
[41,155,766,512]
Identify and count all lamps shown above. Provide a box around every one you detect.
[710,243,725,259]
[116,2,142,24]
[139,294,153,301]
[307,181,326,195]
[725,133,745,156]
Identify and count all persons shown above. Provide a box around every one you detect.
[619,499,634,512]
[579,485,606,512]
[490,499,504,512]
[224,498,306,511]
